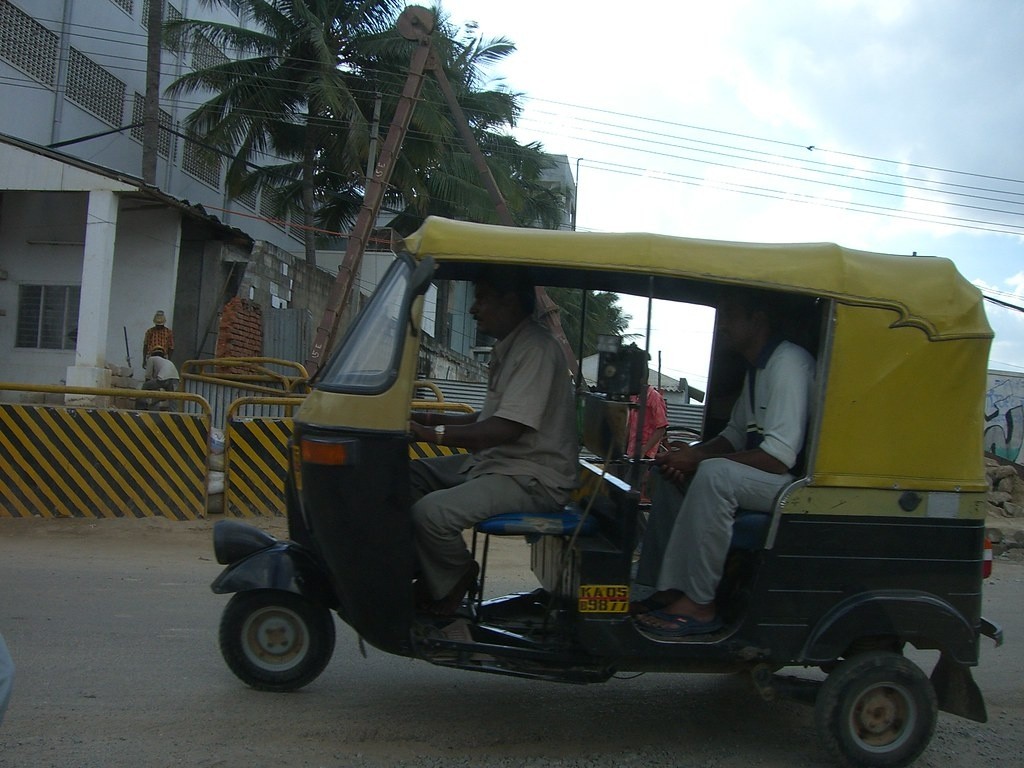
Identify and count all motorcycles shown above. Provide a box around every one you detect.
[211,214,1006,768]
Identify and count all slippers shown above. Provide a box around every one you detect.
[630,596,672,615]
[636,608,723,637]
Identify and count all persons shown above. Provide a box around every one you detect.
[631,288,817,636]
[409,264,579,616]
[142,312,174,369]
[625,371,668,489]
[136,347,180,412]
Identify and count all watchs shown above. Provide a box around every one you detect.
[434,425,445,444]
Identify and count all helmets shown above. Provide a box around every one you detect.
[154,311,166,325]
[152,346,165,354]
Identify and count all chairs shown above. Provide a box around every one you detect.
[469,393,635,646]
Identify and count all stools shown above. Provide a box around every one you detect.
[674,469,772,551]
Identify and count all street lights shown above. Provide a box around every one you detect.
[573,157,584,230]
